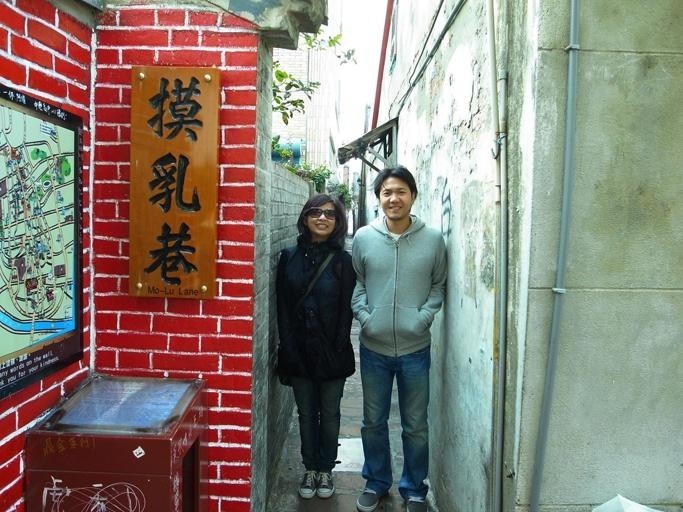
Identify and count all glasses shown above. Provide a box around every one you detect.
[305,206,338,220]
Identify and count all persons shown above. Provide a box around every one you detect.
[349,165,446,511]
[277,194,356,500]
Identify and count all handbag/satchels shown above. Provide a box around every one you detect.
[269,342,280,371]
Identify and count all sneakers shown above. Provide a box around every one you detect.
[316,472,334,499]
[406,498,429,512]
[299,470,316,499]
[356,487,380,512]
